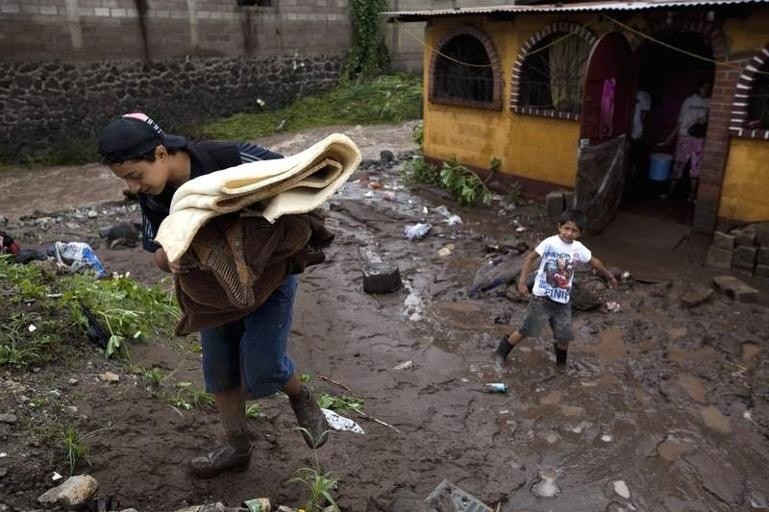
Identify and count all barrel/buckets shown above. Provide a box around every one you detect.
[648,144,674,182]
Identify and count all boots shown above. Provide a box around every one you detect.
[554,347,568,371]
[658,176,696,202]
[493,333,516,370]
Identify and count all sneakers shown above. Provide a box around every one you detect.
[288,382,329,450]
[188,440,254,477]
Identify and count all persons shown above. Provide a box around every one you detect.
[493,208,618,373]
[660,79,712,205]
[95,111,331,480]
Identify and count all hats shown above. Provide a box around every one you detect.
[96,111,188,165]
